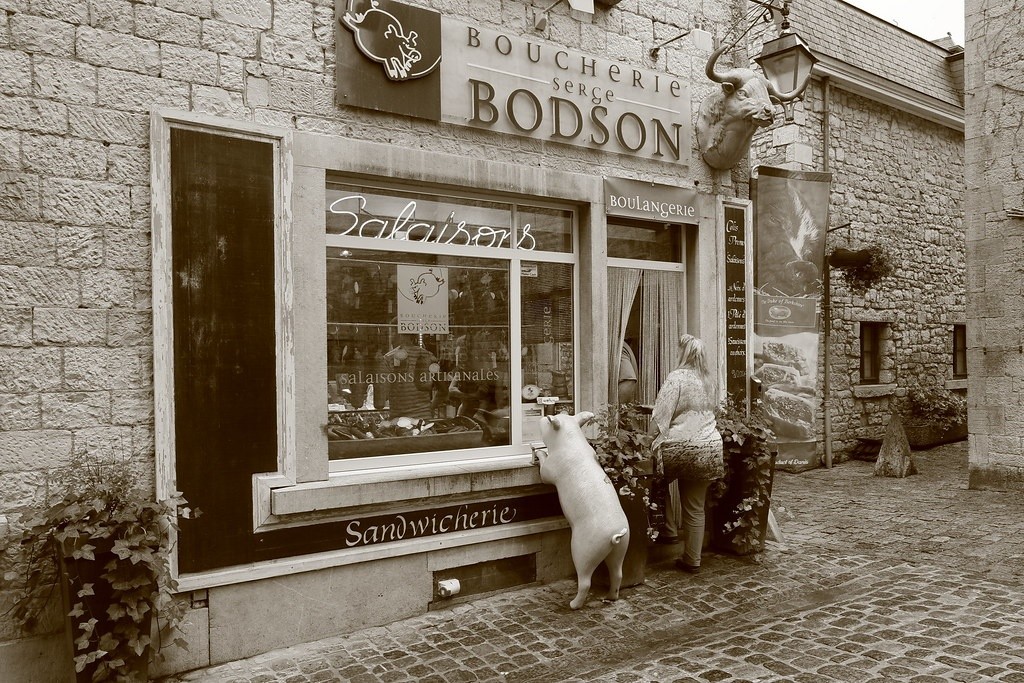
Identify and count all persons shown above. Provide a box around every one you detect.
[653,332,725,573]
[618,338,639,429]
[374,320,495,423]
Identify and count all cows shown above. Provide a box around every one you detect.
[696,45,810,171]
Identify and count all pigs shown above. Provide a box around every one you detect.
[534,410,630,612]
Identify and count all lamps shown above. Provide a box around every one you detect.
[724,0,819,105]
[651,29,711,58]
[535,0,595,31]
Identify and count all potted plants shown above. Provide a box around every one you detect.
[711,414,777,556]
[901,376,976,447]
[585,402,656,590]
[7,472,203,683]
[827,244,892,295]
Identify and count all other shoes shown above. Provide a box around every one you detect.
[675,558,700,574]
[656,534,680,545]
